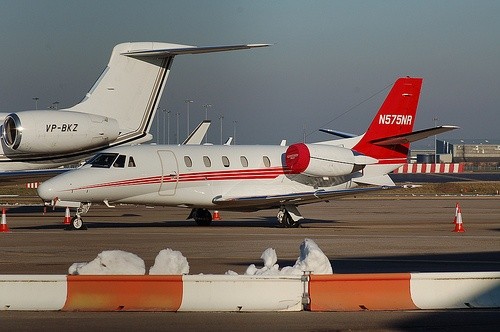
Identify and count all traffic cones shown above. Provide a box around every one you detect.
[451,207,465,233]
[212,210,222,221]
[0,208,10,233]
[451,201,462,224]
[64,206,72,225]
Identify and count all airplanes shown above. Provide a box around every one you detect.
[0,40,272,171]
[35,76,465,230]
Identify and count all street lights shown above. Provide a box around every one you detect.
[155,98,239,145]
